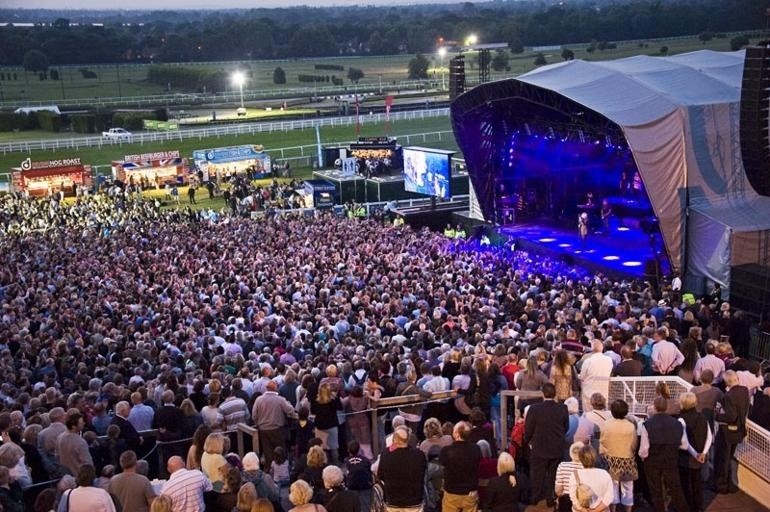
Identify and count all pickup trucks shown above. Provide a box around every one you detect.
[102,127,132,140]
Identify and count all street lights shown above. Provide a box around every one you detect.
[438,46,448,90]
[234,70,247,108]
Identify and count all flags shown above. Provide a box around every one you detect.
[355,94,361,135]
[383,95,394,134]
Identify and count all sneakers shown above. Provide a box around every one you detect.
[716,488,727,494]
[729,485,737,493]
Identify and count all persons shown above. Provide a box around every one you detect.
[1,153,770,512]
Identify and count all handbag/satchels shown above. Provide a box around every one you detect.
[576,483,586,508]
